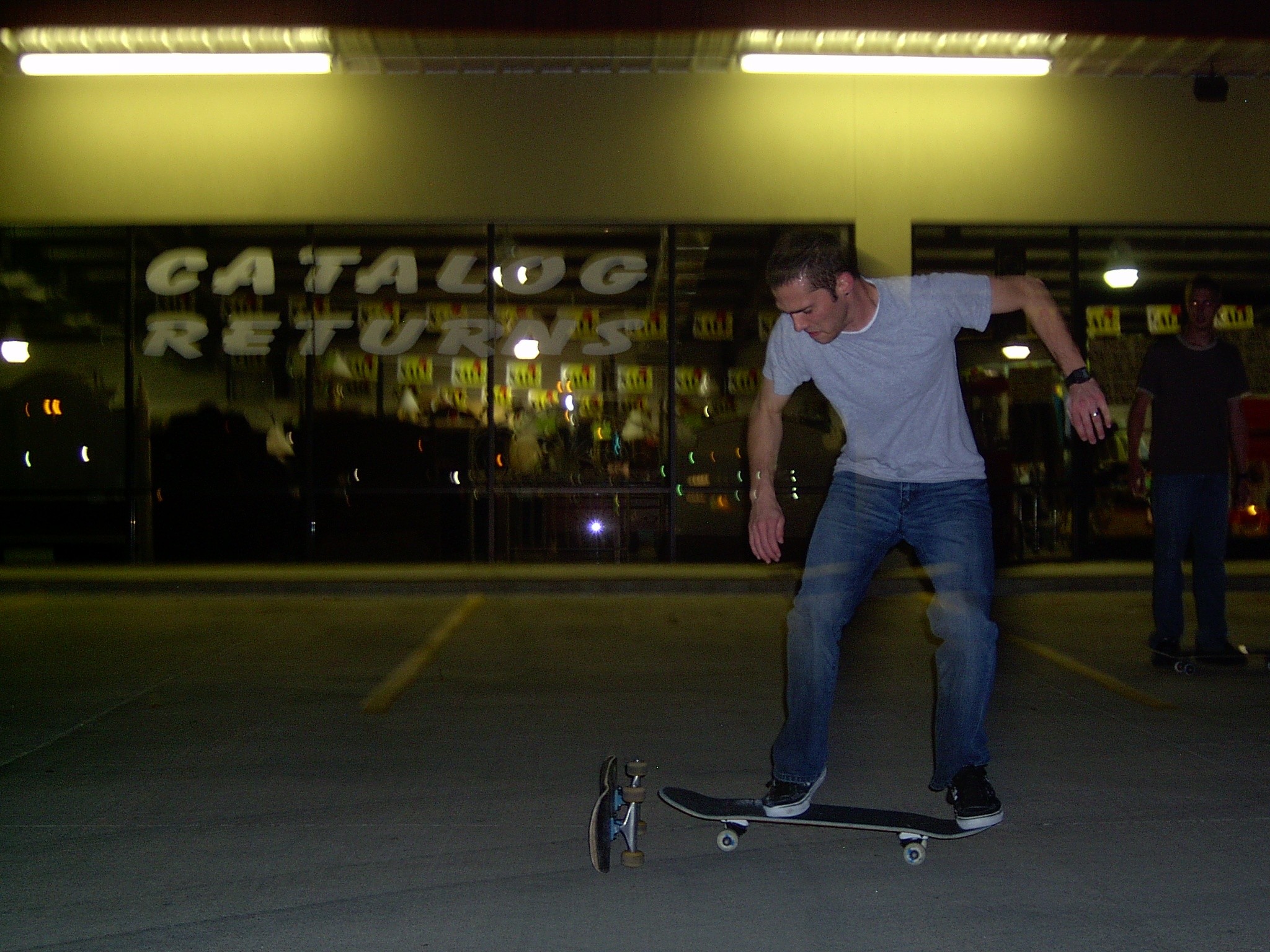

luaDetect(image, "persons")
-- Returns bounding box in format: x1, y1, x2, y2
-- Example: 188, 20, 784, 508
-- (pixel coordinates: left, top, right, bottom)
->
747, 227, 1112, 830
1127, 274, 1252, 662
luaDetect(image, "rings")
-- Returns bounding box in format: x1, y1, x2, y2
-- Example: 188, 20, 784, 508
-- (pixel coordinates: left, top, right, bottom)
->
1091, 412, 1099, 418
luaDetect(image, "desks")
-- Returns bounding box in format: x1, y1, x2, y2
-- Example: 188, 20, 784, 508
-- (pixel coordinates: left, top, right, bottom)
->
495, 472, 665, 564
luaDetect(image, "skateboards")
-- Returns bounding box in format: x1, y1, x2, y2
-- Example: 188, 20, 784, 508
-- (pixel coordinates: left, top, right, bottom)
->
1147, 644, 1270, 675
657, 787, 993, 865
589, 757, 648, 873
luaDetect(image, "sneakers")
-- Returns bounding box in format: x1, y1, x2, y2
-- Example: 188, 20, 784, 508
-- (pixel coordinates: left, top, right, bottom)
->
763, 762, 827, 818
949, 771, 1004, 829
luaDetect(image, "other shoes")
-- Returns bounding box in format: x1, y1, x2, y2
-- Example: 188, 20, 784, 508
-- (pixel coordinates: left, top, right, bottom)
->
1194, 640, 1245, 666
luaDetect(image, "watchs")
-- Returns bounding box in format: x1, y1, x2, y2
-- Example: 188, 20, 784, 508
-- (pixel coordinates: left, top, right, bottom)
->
1064, 366, 1096, 389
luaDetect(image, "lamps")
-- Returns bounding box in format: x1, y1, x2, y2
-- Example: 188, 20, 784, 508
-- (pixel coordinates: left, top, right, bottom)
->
20, 52, 331, 77
1001, 333, 1031, 360
0, 311, 31, 363
740, 54, 1052, 77
1103, 234, 1139, 289
